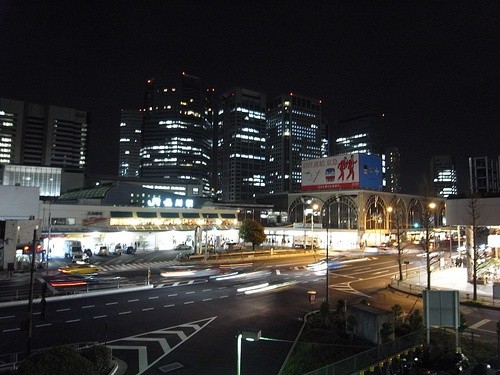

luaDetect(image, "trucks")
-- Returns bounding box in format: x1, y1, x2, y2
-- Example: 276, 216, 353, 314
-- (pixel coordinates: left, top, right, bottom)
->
64, 240, 82, 257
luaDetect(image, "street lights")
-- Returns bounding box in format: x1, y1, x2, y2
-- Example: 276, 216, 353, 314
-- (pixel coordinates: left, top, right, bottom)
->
233, 328, 261, 374
302, 194, 359, 325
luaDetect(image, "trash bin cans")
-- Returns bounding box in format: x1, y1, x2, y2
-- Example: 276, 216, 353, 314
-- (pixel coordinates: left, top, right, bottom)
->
493, 282, 500, 300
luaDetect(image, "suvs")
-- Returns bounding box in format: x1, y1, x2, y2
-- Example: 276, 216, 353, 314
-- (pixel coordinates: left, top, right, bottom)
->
225, 243, 243, 251
122, 246, 133, 254
108, 244, 122, 255
93, 246, 107, 256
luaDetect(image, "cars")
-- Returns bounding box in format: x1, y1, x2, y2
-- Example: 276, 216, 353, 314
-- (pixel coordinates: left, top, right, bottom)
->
71, 253, 90, 262
58, 261, 99, 274
175, 244, 191, 250
199, 245, 213, 253
